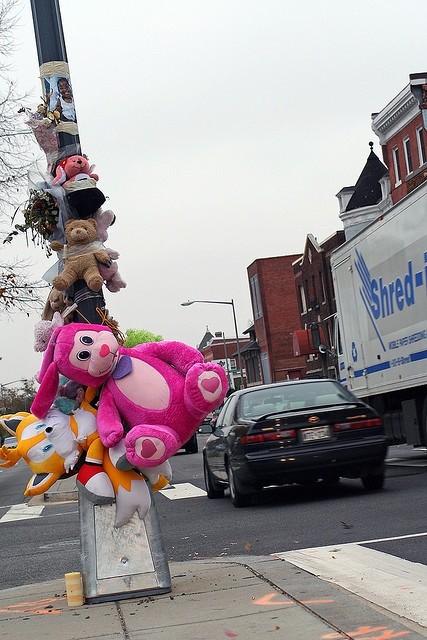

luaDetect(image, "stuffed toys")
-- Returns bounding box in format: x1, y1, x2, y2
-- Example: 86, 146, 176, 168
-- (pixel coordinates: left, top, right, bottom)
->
51, 154, 106, 213
49, 218, 110, 292
33, 311, 65, 384
39, 287, 70, 321
0, 386, 173, 528
53, 374, 85, 415
29, 323, 229, 467
92, 208, 127, 294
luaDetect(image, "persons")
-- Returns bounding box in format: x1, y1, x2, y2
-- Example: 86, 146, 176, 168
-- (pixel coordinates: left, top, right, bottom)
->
56, 77, 77, 122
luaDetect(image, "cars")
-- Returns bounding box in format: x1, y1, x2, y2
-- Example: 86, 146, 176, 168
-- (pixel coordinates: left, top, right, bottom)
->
197, 378, 386, 508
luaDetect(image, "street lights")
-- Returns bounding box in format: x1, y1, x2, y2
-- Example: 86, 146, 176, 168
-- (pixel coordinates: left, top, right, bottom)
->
181, 299, 246, 388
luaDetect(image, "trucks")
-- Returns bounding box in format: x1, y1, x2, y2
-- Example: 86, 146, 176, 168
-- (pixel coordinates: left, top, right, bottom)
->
306, 179, 426, 449
